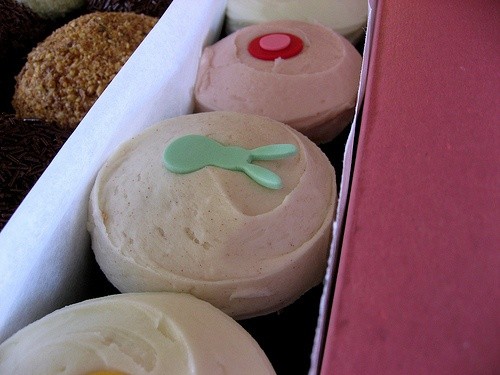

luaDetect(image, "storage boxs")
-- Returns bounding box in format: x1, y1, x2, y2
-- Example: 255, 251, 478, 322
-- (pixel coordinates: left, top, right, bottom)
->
0, 0, 500, 375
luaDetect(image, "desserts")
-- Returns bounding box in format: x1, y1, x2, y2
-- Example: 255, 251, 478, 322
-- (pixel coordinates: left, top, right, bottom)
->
0, 0, 374, 375
